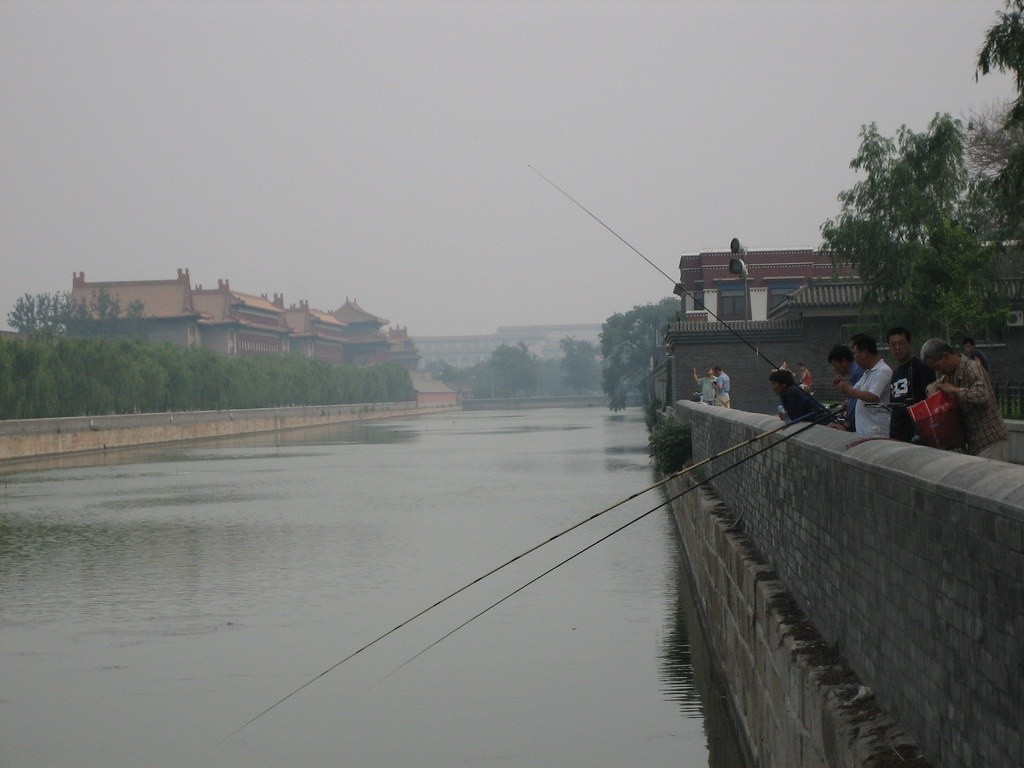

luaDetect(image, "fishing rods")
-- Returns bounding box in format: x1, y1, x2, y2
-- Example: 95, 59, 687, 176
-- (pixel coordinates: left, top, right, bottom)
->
526, 163, 850, 431
363, 402, 847, 694
226, 399, 844, 741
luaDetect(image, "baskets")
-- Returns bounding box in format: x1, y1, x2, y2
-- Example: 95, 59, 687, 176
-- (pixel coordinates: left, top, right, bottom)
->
914, 406, 965, 449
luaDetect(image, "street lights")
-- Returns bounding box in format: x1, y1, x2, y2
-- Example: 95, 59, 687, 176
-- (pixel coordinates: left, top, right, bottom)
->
728, 236, 750, 330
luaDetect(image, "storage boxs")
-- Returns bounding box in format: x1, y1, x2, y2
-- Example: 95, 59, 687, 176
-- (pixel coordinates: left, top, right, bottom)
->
908, 390, 966, 448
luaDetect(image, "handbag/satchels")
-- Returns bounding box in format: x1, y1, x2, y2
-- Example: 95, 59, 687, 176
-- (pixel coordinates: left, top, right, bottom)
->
906, 389, 967, 450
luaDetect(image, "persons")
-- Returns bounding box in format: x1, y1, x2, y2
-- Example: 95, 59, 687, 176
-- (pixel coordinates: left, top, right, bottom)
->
768, 370, 835, 426
889, 328, 937, 446
779, 362, 813, 389
691, 366, 730, 408
920, 336, 1009, 463
829, 333, 893, 438
